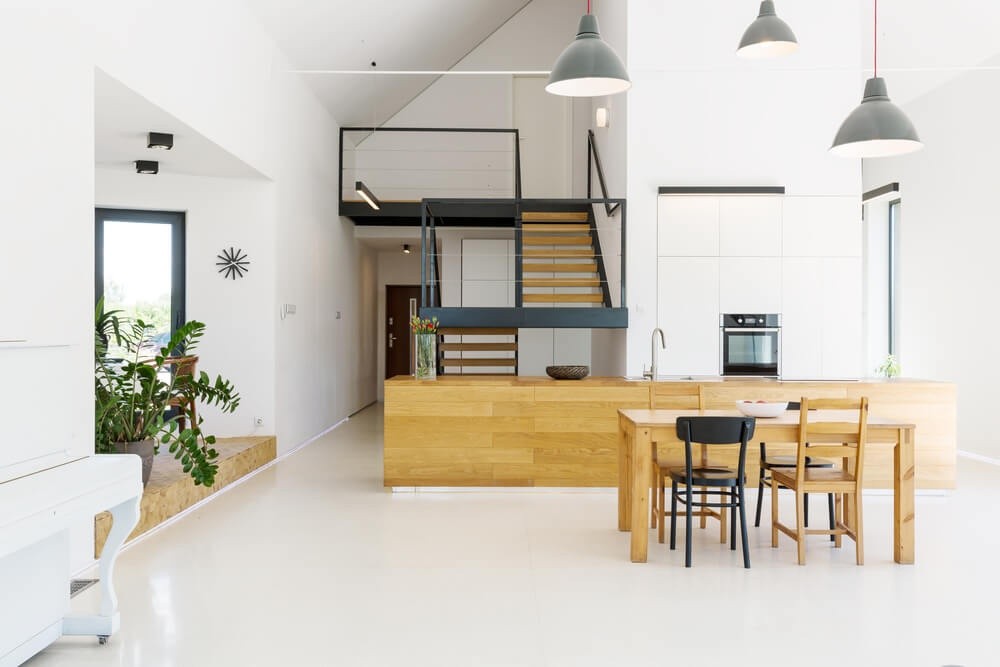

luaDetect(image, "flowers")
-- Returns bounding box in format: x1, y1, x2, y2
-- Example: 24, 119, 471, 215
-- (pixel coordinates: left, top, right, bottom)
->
409, 315, 440, 334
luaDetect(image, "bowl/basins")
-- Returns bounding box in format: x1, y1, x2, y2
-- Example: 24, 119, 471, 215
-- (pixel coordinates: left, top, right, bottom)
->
736, 400, 789, 417
546, 365, 589, 379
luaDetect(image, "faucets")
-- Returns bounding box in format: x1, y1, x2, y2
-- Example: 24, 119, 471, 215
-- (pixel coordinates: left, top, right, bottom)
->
643, 327, 666, 378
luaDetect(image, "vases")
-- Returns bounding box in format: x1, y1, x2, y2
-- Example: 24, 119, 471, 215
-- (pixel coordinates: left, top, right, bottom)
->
414, 335, 437, 379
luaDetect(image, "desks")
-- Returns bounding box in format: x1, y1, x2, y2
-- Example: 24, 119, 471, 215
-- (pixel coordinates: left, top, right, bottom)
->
616, 408, 917, 565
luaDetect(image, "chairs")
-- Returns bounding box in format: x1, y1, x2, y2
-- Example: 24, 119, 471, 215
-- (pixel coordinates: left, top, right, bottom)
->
769, 395, 868, 566
754, 402, 835, 541
670, 416, 756, 569
139, 354, 199, 447
649, 382, 727, 544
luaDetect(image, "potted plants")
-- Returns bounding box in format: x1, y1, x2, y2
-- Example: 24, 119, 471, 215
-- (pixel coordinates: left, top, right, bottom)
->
95, 297, 241, 491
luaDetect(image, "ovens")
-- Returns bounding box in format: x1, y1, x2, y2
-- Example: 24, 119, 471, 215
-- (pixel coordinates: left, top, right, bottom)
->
720, 314, 780, 376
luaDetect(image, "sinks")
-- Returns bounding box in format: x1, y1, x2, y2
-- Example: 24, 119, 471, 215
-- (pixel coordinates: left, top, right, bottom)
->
647, 375, 695, 380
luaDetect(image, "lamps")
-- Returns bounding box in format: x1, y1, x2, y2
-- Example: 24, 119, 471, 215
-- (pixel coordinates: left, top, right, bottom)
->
146, 132, 174, 150
737, 0, 797, 51
356, 182, 380, 210
136, 160, 159, 175
831, 0, 921, 157
545, 0, 630, 97
